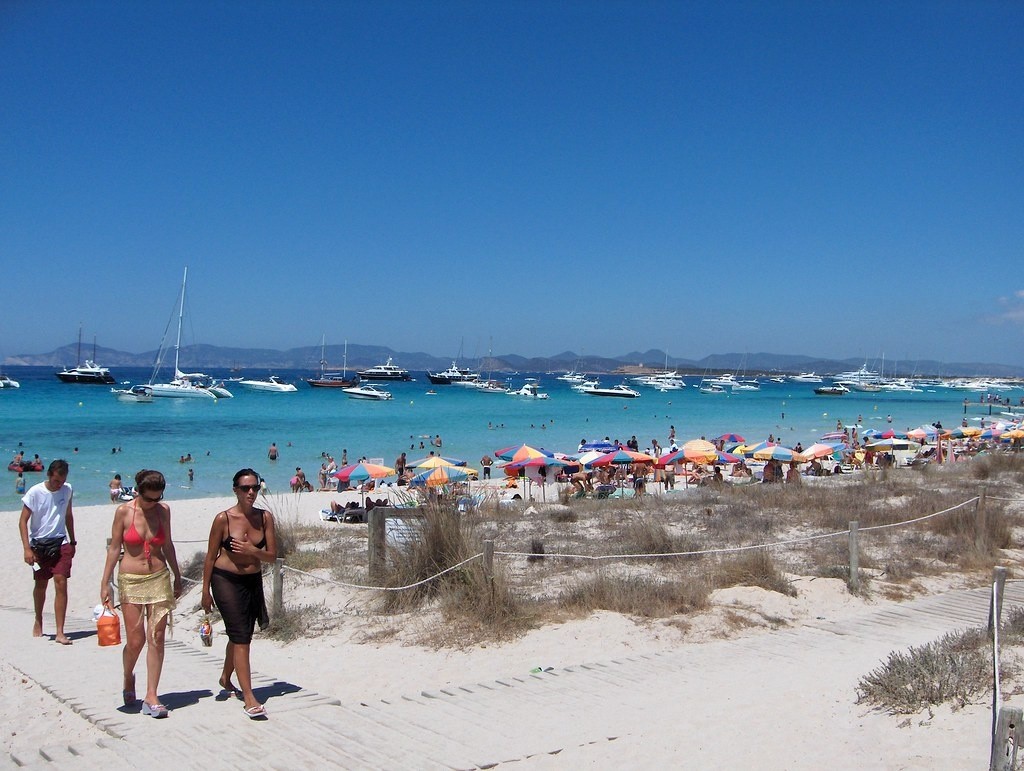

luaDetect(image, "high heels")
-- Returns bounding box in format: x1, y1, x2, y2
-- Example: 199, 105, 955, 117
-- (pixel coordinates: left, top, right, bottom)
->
122, 670, 136, 706
142, 700, 167, 718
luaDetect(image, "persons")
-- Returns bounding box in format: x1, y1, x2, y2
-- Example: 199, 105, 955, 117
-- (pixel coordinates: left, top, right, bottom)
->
35, 455, 41, 465
202, 468, 277, 718
108, 474, 125, 504
14, 451, 24, 464
267, 442, 279, 460
18, 460, 75, 645
188, 469, 194, 481
290, 467, 313, 493
319, 415, 988, 513
179, 453, 191, 464
260, 478, 268, 495
981, 392, 1002, 404
16, 474, 25, 494
1020, 396, 1024, 406
101, 469, 182, 718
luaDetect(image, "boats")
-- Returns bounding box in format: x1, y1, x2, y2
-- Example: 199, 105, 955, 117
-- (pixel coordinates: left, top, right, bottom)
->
118, 486, 139, 501
0, 375, 20, 388
813, 382, 849, 395
342, 381, 392, 401
584, 384, 642, 398
356, 354, 417, 383
118, 384, 153, 403
214, 376, 244, 382
239, 375, 298, 392
769, 372, 823, 383
7, 460, 44, 473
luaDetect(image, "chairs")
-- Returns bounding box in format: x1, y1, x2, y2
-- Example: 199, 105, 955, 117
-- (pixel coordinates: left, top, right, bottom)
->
595, 485, 616, 500
319, 510, 364, 524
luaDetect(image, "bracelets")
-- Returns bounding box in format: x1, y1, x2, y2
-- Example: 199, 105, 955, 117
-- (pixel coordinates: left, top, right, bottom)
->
70, 541, 77, 545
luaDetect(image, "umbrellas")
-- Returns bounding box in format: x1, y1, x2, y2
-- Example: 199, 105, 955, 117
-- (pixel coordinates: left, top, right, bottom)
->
334, 424, 1024, 507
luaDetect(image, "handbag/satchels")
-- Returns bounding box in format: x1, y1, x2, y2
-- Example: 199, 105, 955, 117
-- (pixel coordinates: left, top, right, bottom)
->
97, 602, 121, 646
31, 536, 67, 562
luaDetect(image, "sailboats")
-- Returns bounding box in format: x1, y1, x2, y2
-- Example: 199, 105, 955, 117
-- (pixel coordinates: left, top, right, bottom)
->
300, 333, 359, 387
554, 346, 603, 392
831, 349, 1024, 392
55, 322, 116, 384
137, 266, 234, 400
625, 347, 686, 390
693, 346, 760, 394
423, 335, 549, 399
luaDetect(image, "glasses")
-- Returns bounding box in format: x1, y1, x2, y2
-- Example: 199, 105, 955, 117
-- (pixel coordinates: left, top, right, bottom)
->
234, 485, 262, 492
141, 491, 164, 502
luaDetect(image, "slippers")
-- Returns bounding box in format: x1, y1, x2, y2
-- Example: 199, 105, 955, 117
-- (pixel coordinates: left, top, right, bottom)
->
220, 686, 242, 696
243, 704, 266, 717
